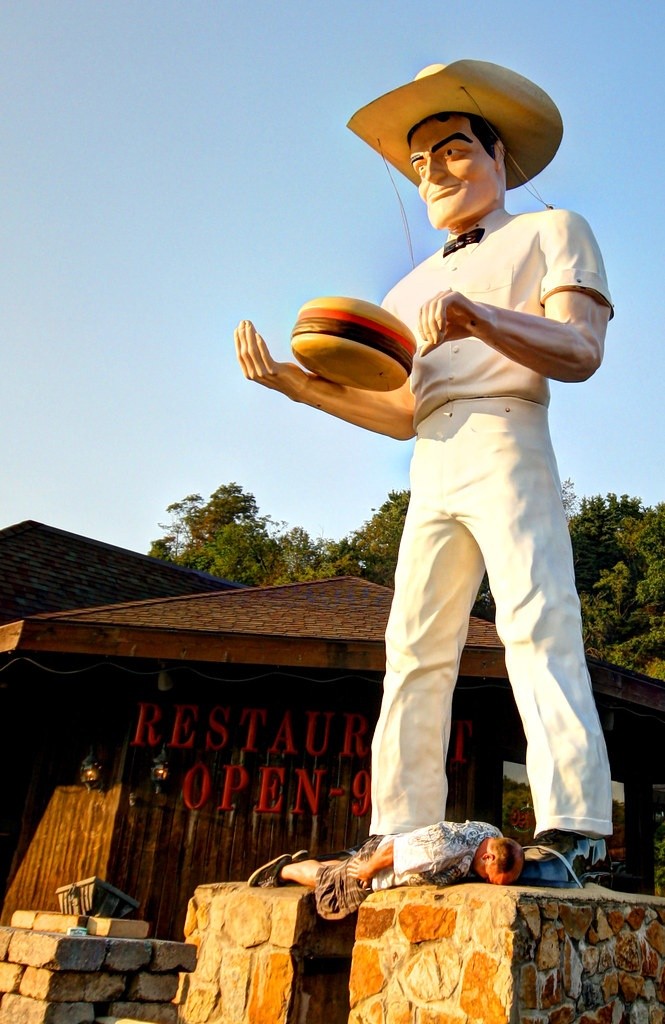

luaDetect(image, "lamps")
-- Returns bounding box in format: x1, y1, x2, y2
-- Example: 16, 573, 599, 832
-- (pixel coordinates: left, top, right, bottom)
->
149, 743, 170, 793
602, 709, 614, 731
79, 748, 111, 794
54, 875, 143, 918
158, 662, 175, 690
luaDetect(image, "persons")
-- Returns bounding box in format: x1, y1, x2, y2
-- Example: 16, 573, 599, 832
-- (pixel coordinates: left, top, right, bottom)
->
234, 61, 611, 885
246, 820, 523, 920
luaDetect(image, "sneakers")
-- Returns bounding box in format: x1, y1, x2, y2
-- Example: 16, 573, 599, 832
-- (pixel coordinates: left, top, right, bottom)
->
247, 853, 293, 888
293, 850, 309, 863
